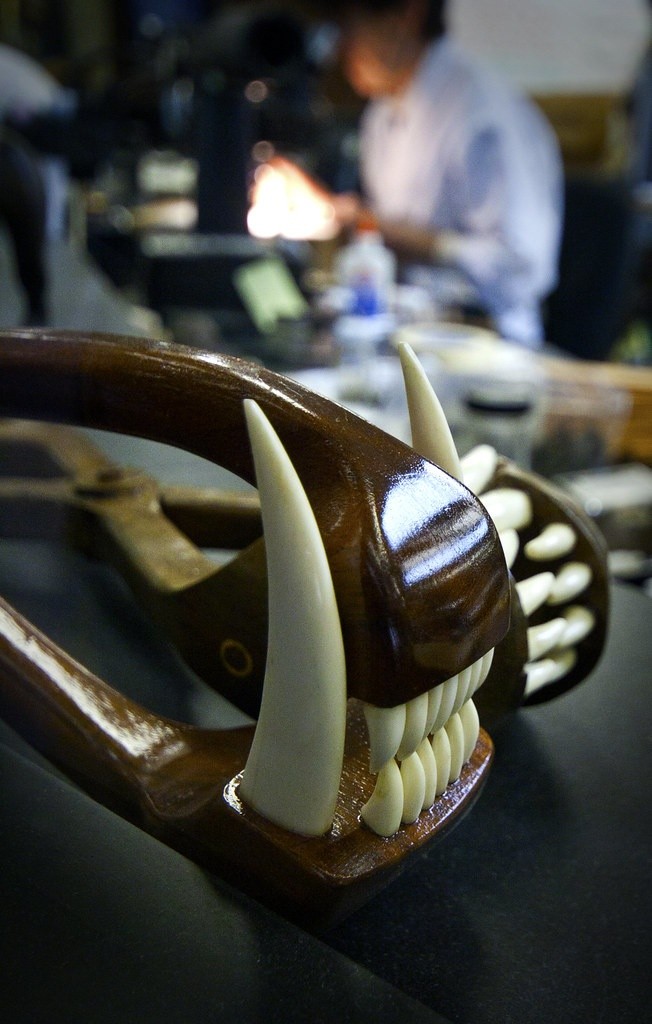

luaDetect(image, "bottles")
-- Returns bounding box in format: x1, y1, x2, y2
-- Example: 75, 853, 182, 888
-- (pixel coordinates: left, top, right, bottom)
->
330, 215, 395, 404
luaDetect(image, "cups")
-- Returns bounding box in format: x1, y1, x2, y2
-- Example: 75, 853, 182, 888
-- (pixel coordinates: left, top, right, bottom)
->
398, 321, 631, 478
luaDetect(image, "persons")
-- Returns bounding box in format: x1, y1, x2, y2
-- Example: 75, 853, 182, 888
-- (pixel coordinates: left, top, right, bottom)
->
333, 0, 563, 350
